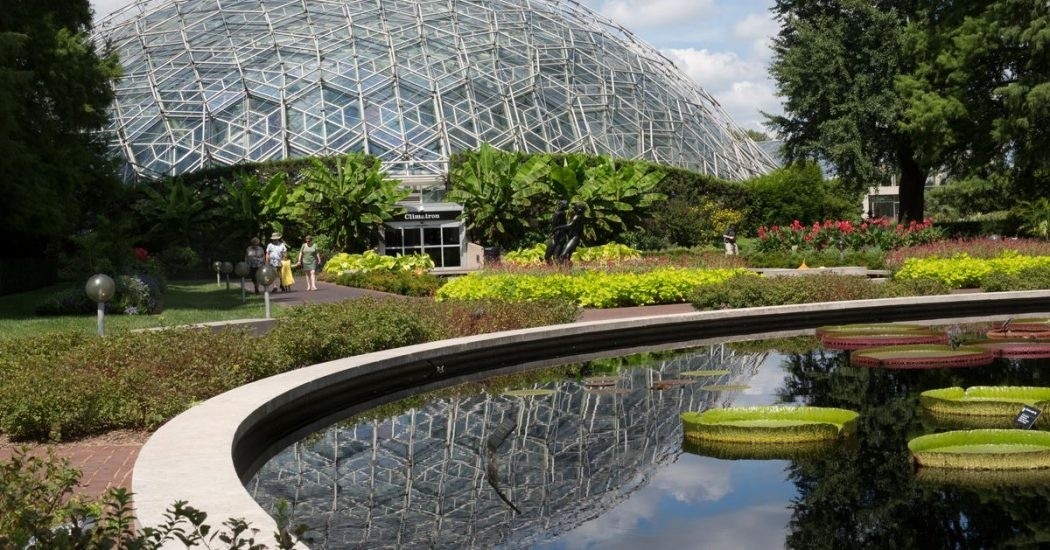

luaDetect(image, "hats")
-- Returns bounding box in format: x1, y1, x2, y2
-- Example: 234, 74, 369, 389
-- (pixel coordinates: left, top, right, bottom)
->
270, 233, 281, 239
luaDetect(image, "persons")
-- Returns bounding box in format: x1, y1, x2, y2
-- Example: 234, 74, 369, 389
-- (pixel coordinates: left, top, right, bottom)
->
544, 200, 587, 272
298, 235, 322, 292
244, 238, 266, 294
278, 252, 298, 293
723, 222, 739, 256
266, 232, 289, 294
